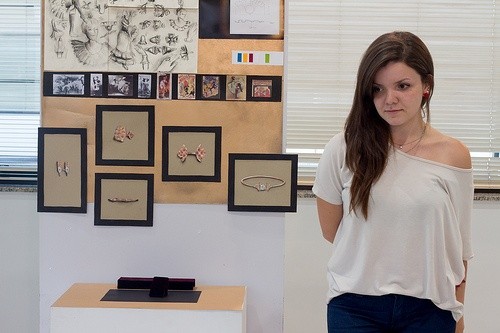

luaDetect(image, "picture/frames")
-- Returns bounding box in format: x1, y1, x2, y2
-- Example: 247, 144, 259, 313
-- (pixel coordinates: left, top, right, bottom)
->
163, 125, 221, 182
95, 173, 155, 227
37, 127, 87, 214
228, 153, 297, 213
95, 105, 154, 166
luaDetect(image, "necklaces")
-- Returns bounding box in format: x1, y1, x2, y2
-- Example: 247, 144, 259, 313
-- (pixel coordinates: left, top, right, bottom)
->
393, 134, 421, 149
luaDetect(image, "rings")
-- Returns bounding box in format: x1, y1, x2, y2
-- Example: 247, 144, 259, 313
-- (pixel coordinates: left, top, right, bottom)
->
241, 174, 285, 192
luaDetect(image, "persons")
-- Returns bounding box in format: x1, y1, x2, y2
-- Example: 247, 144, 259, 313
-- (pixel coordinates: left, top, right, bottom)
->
91, 75, 100, 96
141, 77, 151, 96
312, 31, 473, 332
112, 76, 130, 93
160, 75, 167, 98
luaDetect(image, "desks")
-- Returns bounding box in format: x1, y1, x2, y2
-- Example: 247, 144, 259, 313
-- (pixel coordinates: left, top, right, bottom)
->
49, 284, 247, 332
